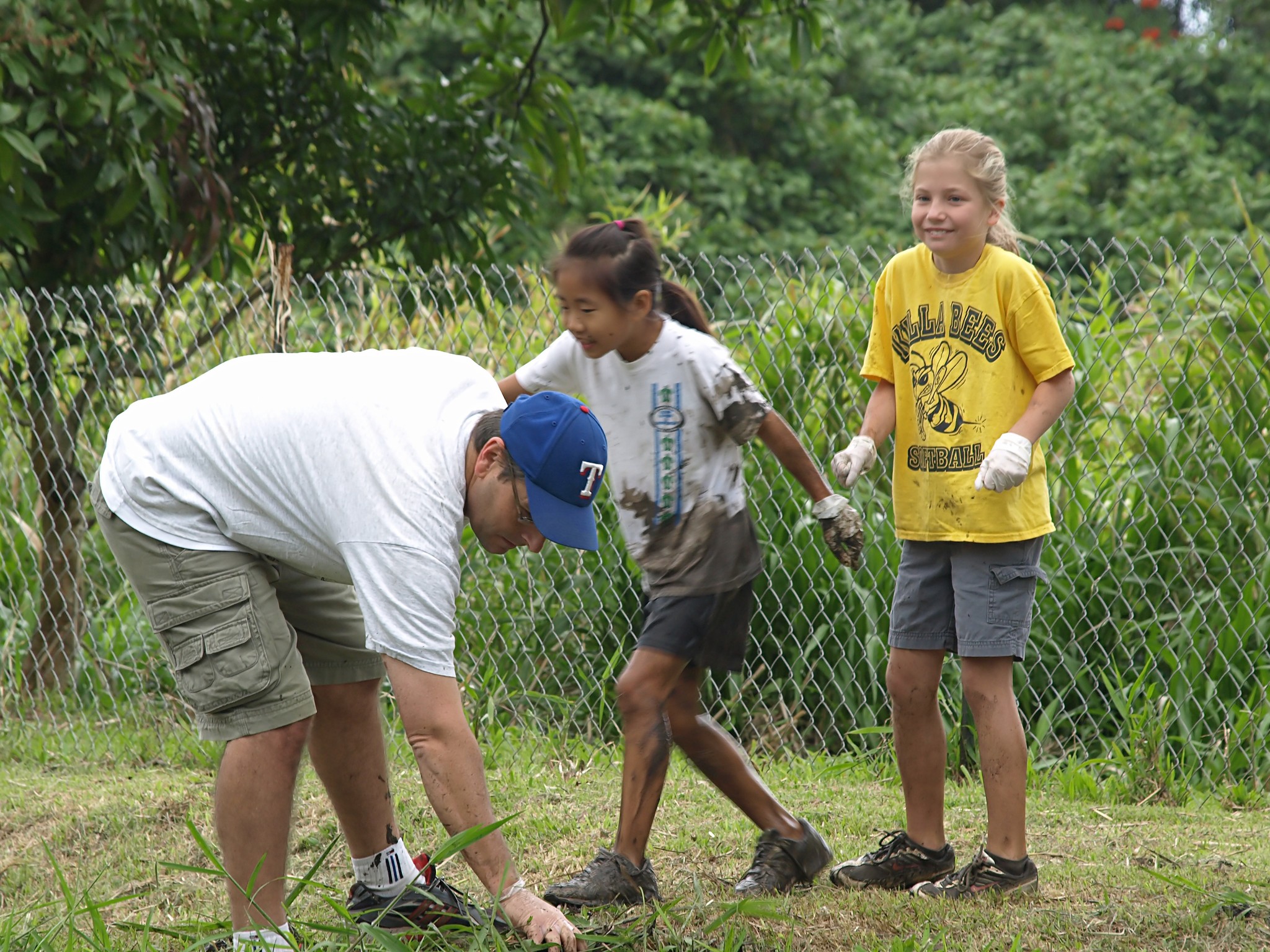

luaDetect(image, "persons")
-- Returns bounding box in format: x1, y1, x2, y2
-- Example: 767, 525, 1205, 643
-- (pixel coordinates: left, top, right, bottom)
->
831, 129, 1074, 901
90, 347, 607, 952
496, 221, 865, 908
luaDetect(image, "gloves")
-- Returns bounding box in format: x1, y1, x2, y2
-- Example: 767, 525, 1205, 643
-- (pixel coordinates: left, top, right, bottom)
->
812, 493, 867, 571
832, 435, 878, 489
976, 433, 1033, 492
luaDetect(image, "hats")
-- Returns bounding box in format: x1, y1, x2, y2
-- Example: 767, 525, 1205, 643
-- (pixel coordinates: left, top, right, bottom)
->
500, 392, 608, 549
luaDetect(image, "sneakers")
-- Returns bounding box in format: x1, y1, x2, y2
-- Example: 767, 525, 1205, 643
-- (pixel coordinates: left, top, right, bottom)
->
739, 819, 833, 895
910, 842, 1041, 901
347, 853, 513, 940
205, 919, 307, 952
545, 851, 660, 907
830, 828, 955, 890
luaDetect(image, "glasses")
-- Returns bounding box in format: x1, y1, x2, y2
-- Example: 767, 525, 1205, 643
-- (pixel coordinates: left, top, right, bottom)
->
504, 450, 535, 528
